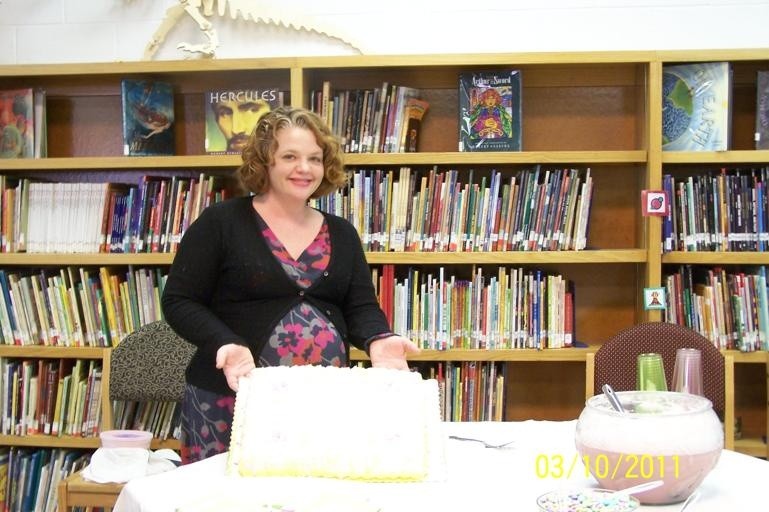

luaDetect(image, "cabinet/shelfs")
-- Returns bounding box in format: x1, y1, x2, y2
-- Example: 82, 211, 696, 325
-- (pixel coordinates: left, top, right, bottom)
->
0, 48, 769, 512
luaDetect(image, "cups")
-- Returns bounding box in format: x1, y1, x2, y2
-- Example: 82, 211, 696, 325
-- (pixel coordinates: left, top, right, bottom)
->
635, 348, 703, 391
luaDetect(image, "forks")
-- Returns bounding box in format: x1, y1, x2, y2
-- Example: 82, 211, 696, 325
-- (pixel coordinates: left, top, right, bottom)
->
450, 435, 515, 448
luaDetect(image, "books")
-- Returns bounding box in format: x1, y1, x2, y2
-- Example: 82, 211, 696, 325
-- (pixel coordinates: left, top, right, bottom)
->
1, 359, 103, 438
0, 266, 168, 347
661, 264, 768, 352
663, 61, 733, 153
1, 447, 104, 512
1, 175, 231, 254
113, 397, 183, 441
661, 166, 768, 252
458, 71, 522, 153
351, 360, 506, 421
1, 88, 48, 159
755, 71, 768, 149
122, 79, 176, 155
205, 91, 283, 155
308, 165, 593, 253
371, 263, 575, 350
308, 80, 427, 153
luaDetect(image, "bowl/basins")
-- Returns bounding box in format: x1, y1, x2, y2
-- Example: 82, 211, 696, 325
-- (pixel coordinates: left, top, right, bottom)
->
101, 429, 153, 449
574, 391, 724, 505
537, 485, 640, 512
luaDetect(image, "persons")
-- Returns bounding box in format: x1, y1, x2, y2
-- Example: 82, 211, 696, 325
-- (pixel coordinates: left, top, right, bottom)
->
160, 107, 420, 466
